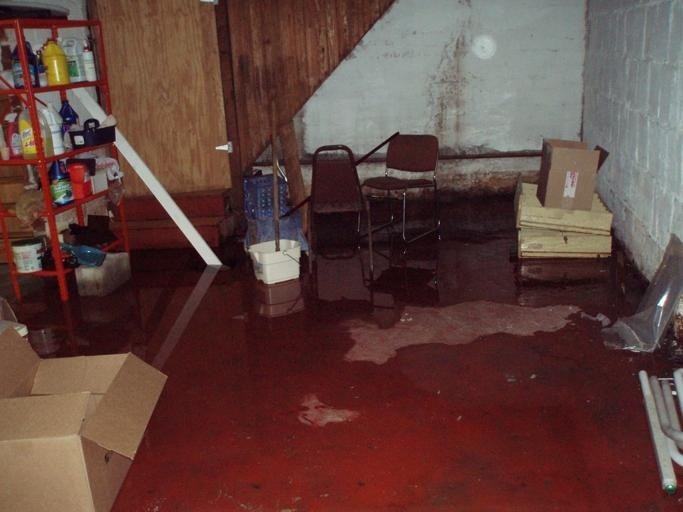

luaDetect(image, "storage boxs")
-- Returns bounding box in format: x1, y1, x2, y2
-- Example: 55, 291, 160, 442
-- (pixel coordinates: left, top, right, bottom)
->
536, 138, 609, 210
0, 326, 170, 510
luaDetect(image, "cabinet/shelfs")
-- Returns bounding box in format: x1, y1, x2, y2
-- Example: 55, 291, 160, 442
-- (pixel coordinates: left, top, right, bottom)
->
0, 18, 130, 306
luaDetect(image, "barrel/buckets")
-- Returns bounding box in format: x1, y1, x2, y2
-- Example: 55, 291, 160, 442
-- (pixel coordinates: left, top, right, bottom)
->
254, 280, 305, 317
29, 328, 60, 355
11, 240, 43, 274
249, 238, 302, 284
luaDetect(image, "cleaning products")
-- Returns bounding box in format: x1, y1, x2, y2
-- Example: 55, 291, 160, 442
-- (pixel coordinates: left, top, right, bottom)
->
0, 37, 96, 207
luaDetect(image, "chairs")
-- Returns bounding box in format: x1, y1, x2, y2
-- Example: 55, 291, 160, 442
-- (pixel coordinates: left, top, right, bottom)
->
305, 134, 444, 288
